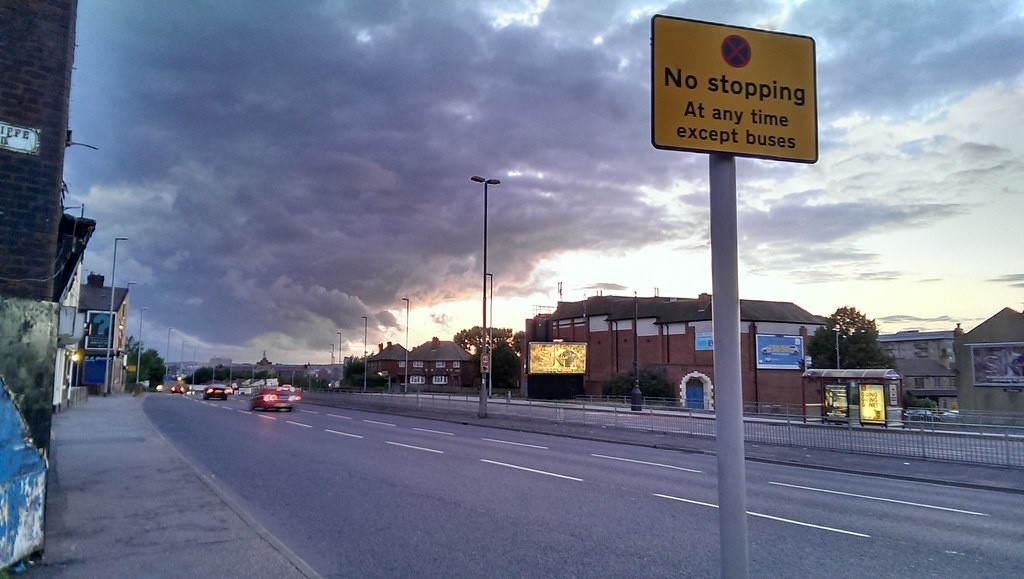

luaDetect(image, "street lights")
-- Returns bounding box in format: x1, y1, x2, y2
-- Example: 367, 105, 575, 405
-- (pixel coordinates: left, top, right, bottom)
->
136, 308, 149, 382
164, 327, 175, 387
193, 349, 198, 389
336, 333, 341, 388
180, 341, 188, 379
402, 298, 410, 393
127, 281, 137, 289
102, 237, 128, 396
486, 273, 493, 397
361, 317, 367, 392
470, 176, 501, 418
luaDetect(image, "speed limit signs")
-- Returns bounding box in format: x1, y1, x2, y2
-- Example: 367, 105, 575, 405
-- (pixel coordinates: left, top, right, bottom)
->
481, 354, 490, 364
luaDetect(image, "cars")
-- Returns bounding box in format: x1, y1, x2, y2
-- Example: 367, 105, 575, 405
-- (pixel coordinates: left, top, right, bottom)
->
171, 385, 183, 395
203, 384, 228, 400
248, 388, 295, 412
904, 410, 941, 427
